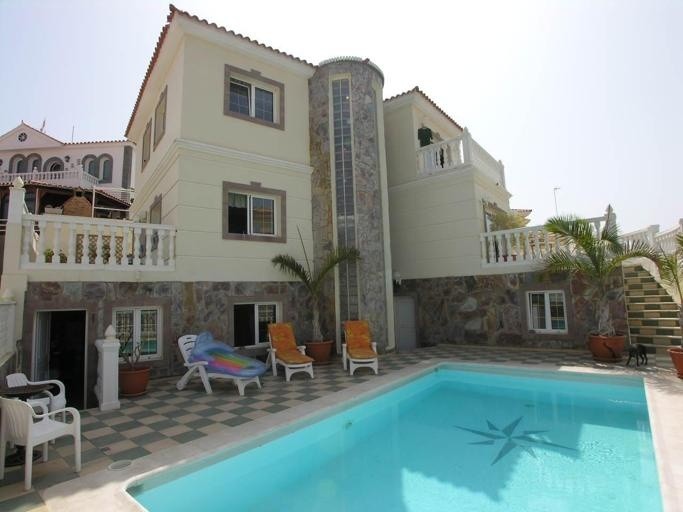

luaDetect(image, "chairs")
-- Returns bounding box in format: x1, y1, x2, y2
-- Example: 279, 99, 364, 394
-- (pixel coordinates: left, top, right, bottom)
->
176, 335, 262, 395
0, 372, 84, 491
340, 318, 380, 378
264, 322, 316, 384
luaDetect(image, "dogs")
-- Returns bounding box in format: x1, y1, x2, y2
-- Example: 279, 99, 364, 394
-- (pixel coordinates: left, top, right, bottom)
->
622, 343, 647, 366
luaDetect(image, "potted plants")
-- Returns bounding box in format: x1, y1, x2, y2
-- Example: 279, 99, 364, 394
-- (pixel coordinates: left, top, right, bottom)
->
116, 327, 151, 395
44, 248, 55, 263
536, 211, 682, 379
268, 246, 364, 365
